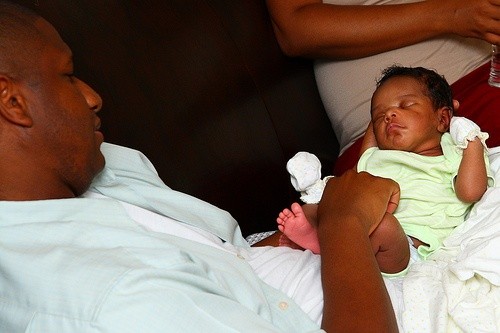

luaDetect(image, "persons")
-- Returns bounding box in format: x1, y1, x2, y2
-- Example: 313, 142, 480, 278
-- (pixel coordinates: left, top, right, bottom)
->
1, 1, 500, 333
268, 1, 500, 181
277, 65, 495, 279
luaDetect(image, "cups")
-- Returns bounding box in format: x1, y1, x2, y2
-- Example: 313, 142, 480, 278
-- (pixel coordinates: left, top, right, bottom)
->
488, 45, 500, 87
